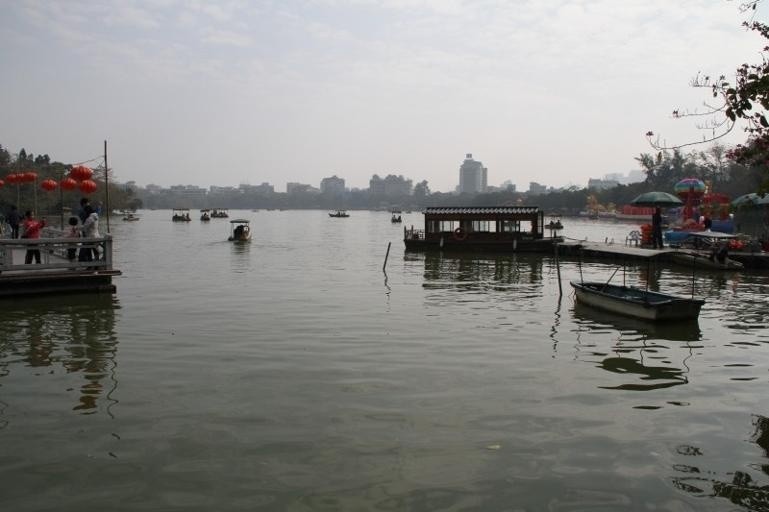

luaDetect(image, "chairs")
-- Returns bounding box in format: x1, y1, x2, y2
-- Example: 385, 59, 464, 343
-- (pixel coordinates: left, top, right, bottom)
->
625, 230, 642, 246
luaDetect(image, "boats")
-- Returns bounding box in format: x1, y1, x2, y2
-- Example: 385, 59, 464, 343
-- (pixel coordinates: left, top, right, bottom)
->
227, 219, 252, 241
200, 208, 210, 222
211, 207, 229, 217
403, 205, 564, 253
123, 211, 140, 222
328, 209, 349, 217
665, 229, 746, 271
391, 210, 402, 223
543, 219, 564, 230
172, 207, 192, 222
569, 278, 706, 321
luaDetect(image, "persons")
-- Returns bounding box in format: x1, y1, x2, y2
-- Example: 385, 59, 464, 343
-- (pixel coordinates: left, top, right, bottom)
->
550, 220, 555, 225
702, 212, 713, 232
651, 207, 665, 249
556, 220, 560, 224
5, 199, 101, 269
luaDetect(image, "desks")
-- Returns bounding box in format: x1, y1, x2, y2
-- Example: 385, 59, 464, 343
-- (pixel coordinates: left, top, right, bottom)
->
689, 231, 738, 252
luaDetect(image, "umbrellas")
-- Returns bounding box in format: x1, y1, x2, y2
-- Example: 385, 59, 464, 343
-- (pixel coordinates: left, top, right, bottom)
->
629, 191, 683, 225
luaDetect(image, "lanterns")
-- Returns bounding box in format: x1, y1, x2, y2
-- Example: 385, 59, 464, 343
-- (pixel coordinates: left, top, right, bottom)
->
0, 165, 96, 194
